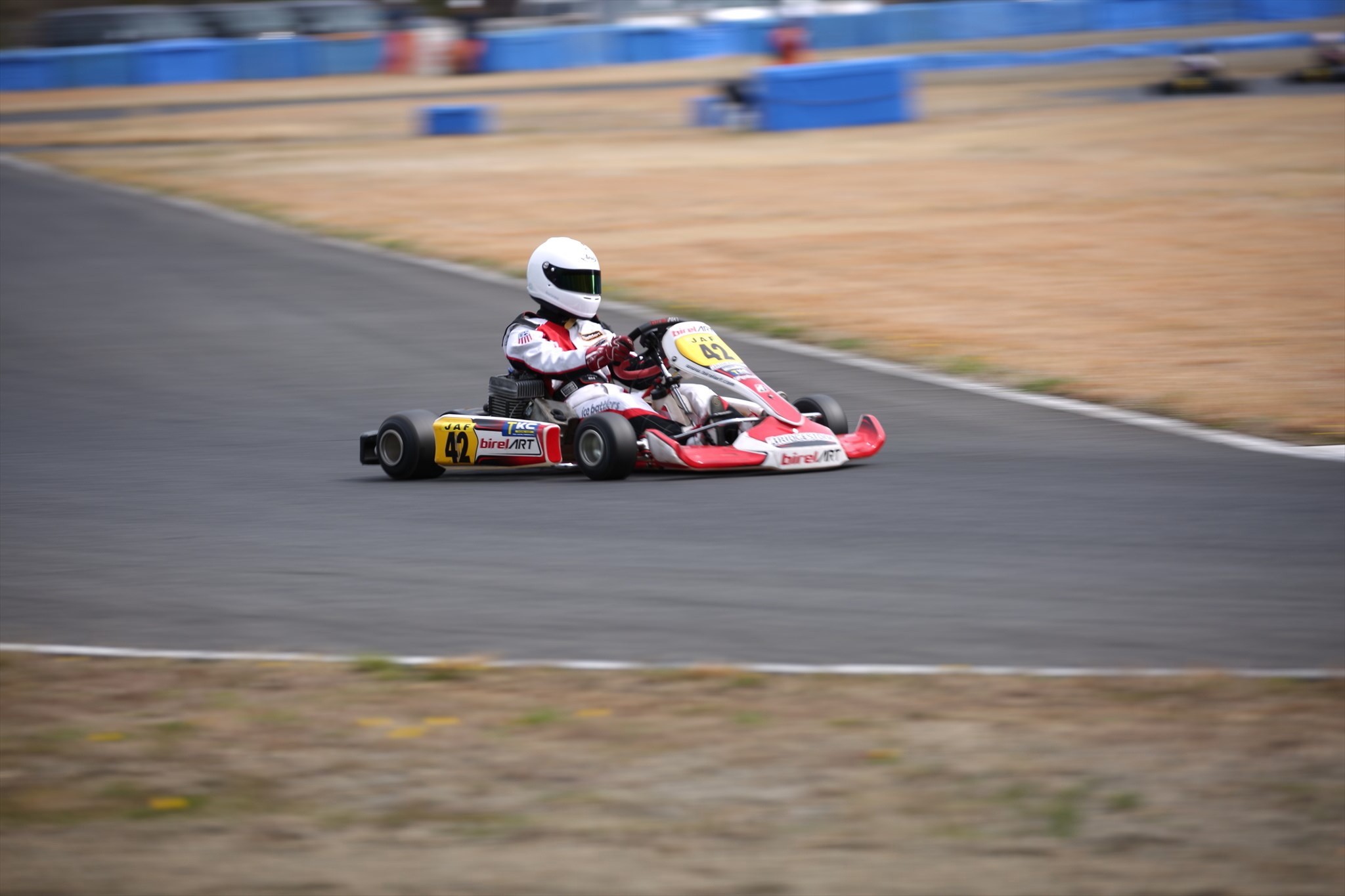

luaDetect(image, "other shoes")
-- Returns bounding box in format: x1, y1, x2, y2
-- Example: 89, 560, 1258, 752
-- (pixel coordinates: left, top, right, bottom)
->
700, 395, 726, 446
766, 392, 788, 416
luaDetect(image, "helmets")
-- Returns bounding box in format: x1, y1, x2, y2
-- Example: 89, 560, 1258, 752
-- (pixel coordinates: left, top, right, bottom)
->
527, 237, 601, 320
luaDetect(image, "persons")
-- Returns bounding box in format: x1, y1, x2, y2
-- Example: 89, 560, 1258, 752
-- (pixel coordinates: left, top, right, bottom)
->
502, 236, 752, 445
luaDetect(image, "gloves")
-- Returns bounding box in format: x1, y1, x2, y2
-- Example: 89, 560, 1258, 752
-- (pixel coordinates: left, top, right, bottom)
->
585, 336, 634, 374
